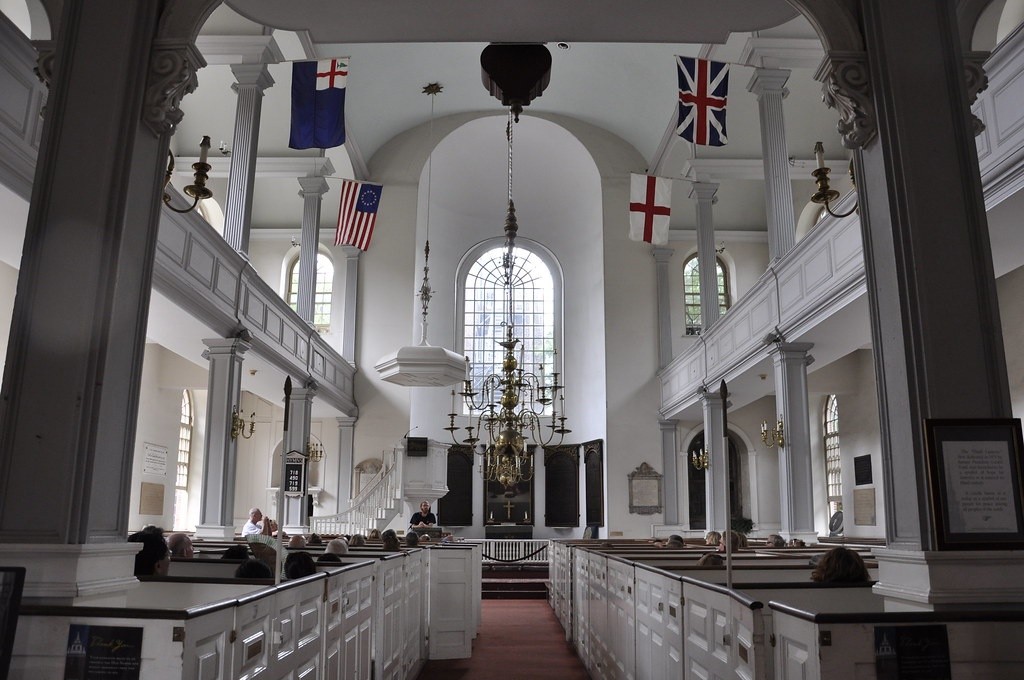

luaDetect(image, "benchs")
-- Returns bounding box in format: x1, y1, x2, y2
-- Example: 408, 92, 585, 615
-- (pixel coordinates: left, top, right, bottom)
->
548, 539, 881, 680
135, 535, 485, 680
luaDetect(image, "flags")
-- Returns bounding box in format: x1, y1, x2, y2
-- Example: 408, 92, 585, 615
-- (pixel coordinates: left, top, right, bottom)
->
335, 179, 383, 251
676, 56, 730, 146
288, 57, 349, 150
629, 173, 673, 245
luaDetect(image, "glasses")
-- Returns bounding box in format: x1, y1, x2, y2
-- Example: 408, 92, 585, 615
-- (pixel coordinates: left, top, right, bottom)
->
812, 571, 820, 576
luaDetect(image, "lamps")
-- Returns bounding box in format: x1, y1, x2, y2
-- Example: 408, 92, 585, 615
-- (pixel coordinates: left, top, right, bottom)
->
696, 386, 706, 395
716, 241, 725, 253
375, 84, 472, 389
761, 418, 784, 448
762, 333, 781, 346
242, 329, 254, 342
291, 233, 301, 247
810, 142, 859, 218
781, 88, 790, 100
162, 136, 212, 213
308, 442, 323, 463
310, 381, 319, 391
692, 447, 709, 470
713, 196, 718, 205
442, 41, 575, 500
231, 405, 255, 443
727, 401, 734, 408
219, 140, 231, 158
201, 350, 210, 360
806, 355, 815, 366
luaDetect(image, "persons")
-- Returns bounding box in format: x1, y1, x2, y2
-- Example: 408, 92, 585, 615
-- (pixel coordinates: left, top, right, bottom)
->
128, 525, 169, 579
166, 533, 193, 557
410, 500, 436, 528
241, 508, 272, 537
811, 546, 869, 589
221, 528, 453, 580
658, 529, 806, 567
